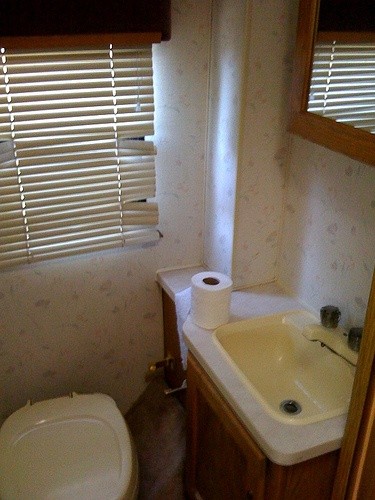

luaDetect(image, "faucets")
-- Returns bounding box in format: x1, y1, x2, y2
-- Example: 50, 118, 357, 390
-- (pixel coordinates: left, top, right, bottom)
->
302, 322, 358, 367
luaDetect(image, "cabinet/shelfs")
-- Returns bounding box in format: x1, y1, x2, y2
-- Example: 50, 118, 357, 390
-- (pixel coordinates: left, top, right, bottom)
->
185, 350, 267, 500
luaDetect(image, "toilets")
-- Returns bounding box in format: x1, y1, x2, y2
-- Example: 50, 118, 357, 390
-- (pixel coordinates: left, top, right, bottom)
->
1, 390, 141, 499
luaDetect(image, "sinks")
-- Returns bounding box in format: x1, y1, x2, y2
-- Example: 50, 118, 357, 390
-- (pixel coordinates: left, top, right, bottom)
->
212, 309, 358, 426
